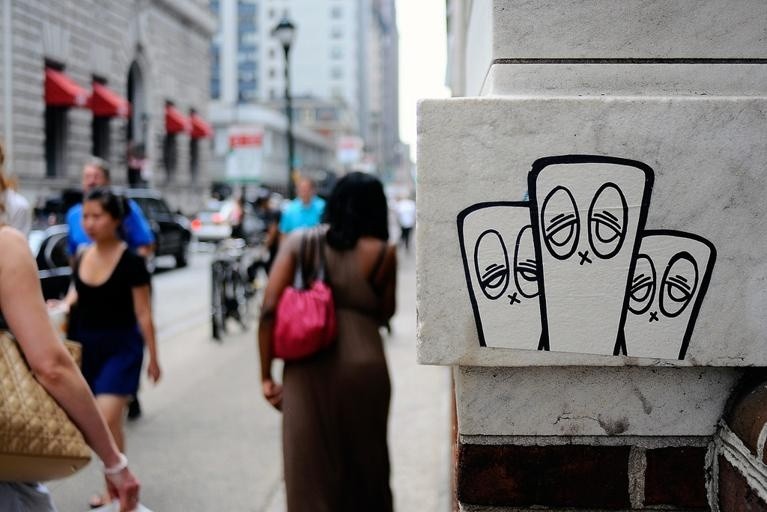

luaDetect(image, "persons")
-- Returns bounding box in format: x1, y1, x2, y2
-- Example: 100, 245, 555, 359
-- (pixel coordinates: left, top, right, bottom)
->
1, 136, 144, 512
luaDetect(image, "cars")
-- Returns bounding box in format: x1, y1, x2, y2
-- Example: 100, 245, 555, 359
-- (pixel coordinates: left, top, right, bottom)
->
194, 202, 241, 242
124, 188, 195, 267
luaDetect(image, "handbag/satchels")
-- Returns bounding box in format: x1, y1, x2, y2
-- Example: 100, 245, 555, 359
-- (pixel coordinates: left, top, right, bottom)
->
272, 282, 336, 359
0, 331, 91, 482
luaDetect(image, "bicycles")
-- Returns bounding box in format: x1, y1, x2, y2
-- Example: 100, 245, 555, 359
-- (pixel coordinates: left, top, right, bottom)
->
212, 258, 250, 337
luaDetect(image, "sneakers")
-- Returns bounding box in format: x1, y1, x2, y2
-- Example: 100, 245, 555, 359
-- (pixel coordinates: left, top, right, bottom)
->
127, 404, 142, 421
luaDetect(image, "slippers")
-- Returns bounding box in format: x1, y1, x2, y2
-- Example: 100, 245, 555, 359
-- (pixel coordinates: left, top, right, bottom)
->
89, 492, 111, 507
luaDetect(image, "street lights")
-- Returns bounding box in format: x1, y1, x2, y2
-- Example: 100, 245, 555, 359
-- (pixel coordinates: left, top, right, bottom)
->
271, 11, 297, 196
